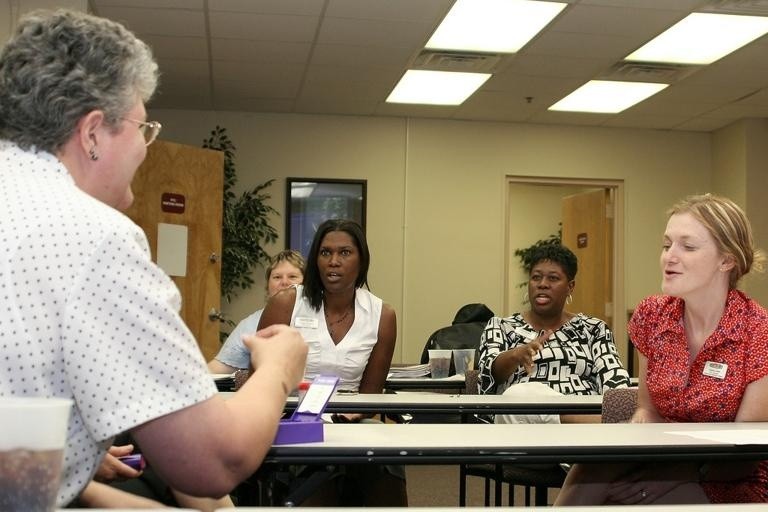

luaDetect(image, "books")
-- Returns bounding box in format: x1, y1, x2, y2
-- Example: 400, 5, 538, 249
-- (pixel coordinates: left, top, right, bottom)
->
389, 363, 431, 379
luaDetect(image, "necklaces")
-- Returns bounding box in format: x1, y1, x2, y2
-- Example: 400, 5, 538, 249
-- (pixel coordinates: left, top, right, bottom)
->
323, 298, 354, 339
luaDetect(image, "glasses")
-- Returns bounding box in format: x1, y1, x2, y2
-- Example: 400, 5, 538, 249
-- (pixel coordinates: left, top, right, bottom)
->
120, 116, 162, 146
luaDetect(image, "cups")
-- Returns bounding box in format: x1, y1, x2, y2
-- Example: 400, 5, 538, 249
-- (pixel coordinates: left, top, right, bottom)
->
428, 349, 452, 380
450, 349, 477, 375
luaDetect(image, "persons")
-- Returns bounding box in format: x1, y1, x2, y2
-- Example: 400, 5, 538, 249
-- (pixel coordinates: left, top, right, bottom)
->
248, 219, 410, 506
476, 242, 632, 471
63, 429, 235, 510
2, 4, 309, 509
546, 192, 768, 504
205, 249, 308, 375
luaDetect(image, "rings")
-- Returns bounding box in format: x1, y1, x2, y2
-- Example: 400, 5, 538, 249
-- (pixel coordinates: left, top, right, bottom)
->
640, 488, 649, 498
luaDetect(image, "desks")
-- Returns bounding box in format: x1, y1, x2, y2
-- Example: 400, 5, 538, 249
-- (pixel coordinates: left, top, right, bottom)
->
216, 364, 768, 506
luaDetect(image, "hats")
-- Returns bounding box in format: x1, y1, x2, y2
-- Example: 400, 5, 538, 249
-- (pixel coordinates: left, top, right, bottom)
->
452, 304, 494, 325
